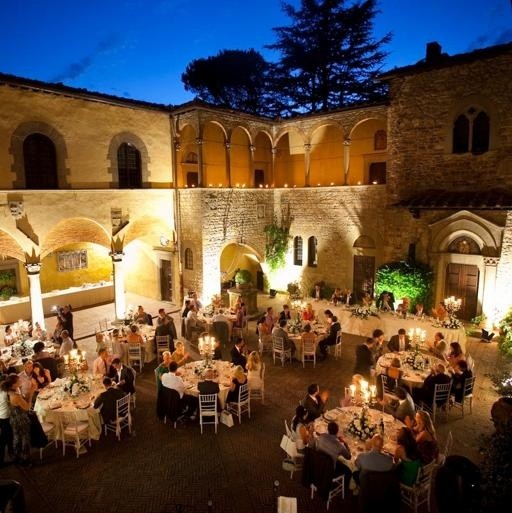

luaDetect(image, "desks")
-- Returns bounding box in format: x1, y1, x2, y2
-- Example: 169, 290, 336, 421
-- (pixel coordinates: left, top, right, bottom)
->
257, 290, 468, 355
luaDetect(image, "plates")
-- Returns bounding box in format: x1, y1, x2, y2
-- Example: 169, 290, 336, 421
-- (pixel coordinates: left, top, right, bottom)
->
324, 411, 337, 420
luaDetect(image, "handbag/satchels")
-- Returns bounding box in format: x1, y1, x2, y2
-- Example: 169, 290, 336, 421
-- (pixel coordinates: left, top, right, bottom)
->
280, 435, 298, 458
219, 410, 234, 427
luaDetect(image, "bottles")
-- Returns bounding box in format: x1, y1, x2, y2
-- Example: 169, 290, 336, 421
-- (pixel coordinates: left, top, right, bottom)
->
379, 418, 384, 434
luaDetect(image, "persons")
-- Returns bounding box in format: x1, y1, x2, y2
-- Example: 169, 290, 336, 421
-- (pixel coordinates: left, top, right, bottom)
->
181, 291, 246, 335
112, 304, 177, 366
255, 303, 341, 363
0, 304, 135, 466
156, 338, 248, 428
311, 282, 449, 324
289, 375, 440, 513
355, 328, 473, 411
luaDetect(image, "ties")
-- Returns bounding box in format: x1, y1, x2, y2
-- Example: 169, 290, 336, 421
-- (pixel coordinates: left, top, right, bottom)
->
103, 359, 107, 376
400, 339, 403, 351
